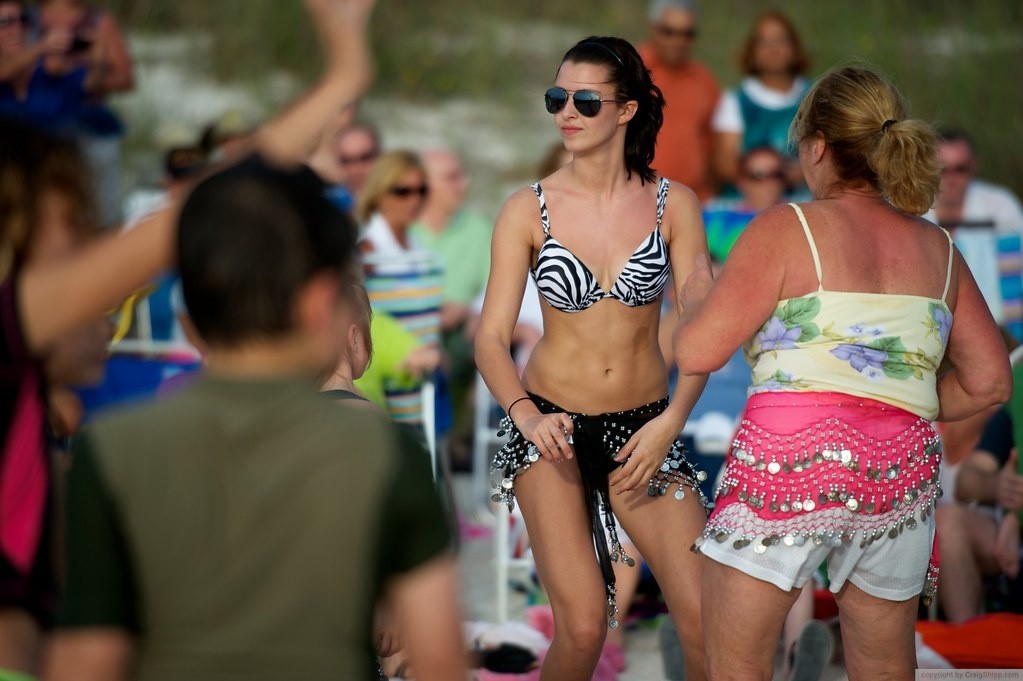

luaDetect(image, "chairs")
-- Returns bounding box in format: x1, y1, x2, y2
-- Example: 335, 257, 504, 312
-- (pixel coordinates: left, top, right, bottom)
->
471, 370, 507, 500
362, 248, 446, 485
494, 492, 534, 623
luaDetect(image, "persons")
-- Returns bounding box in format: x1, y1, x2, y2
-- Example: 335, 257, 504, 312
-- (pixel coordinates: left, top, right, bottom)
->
40, 147, 466, 681
472, 33, 712, 681
0, 0, 374, 681
0, 0, 1023, 681
672, 59, 1012, 681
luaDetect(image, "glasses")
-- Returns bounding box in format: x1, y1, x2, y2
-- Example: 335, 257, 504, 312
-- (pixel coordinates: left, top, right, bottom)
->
544, 87, 630, 118
336, 151, 378, 167
655, 22, 696, 41
0, 14, 35, 28
388, 186, 428, 199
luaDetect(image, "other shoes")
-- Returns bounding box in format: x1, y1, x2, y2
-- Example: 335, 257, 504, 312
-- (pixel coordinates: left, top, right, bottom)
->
788, 619, 833, 681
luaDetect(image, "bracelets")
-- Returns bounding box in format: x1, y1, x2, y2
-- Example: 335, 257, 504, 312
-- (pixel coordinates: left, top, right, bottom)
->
508, 397, 533, 418
